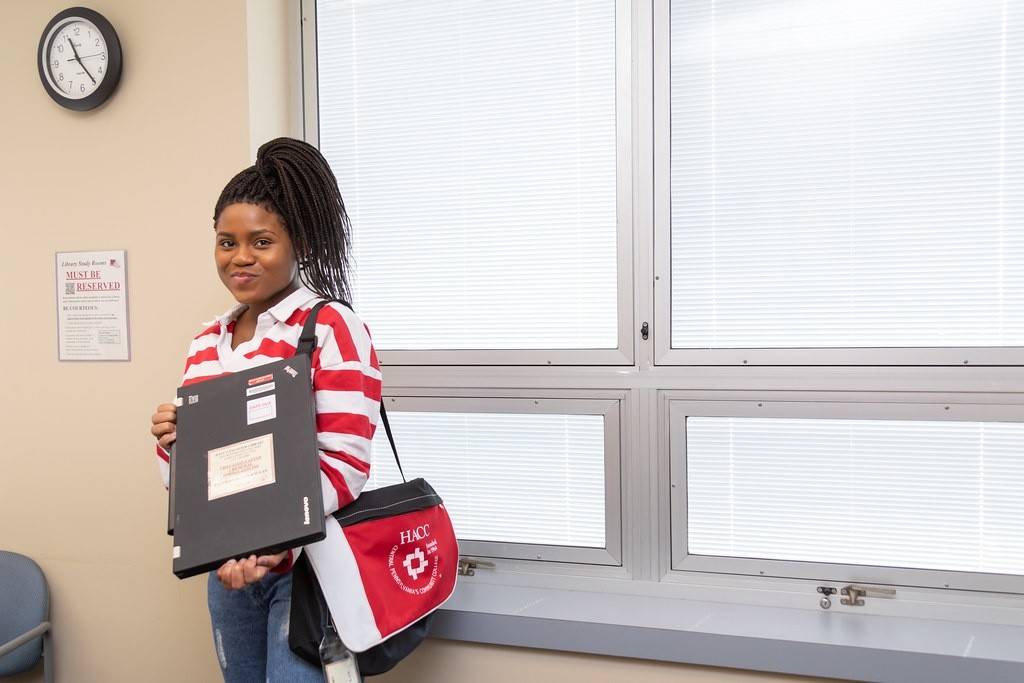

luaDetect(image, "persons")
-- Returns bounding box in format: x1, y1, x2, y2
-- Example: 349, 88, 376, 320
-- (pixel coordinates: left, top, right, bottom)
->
151, 137, 381, 683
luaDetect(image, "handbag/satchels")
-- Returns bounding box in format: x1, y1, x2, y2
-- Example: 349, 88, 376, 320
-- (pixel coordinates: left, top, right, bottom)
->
287, 476, 460, 678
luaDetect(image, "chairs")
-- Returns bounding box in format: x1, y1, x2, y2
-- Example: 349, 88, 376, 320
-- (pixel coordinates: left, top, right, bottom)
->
0, 551, 52, 683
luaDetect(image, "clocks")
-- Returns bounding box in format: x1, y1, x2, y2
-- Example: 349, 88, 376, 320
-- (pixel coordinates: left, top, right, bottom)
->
37, 6, 123, 112
